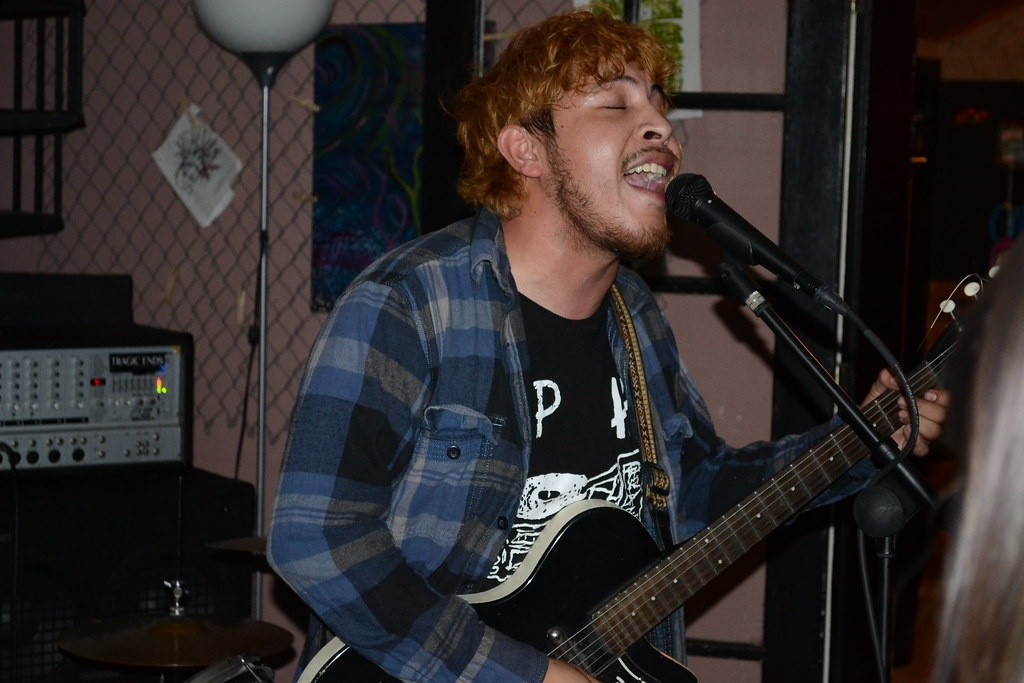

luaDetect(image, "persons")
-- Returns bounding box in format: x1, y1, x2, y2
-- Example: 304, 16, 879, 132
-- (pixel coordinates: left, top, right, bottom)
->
266, 2, 1023, 683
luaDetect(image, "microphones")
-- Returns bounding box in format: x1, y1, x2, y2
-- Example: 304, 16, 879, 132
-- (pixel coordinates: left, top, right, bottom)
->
666, 172, 843, 312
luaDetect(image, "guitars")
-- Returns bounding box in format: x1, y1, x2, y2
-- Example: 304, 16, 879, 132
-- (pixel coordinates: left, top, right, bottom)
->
293, 260, 1013, 683
